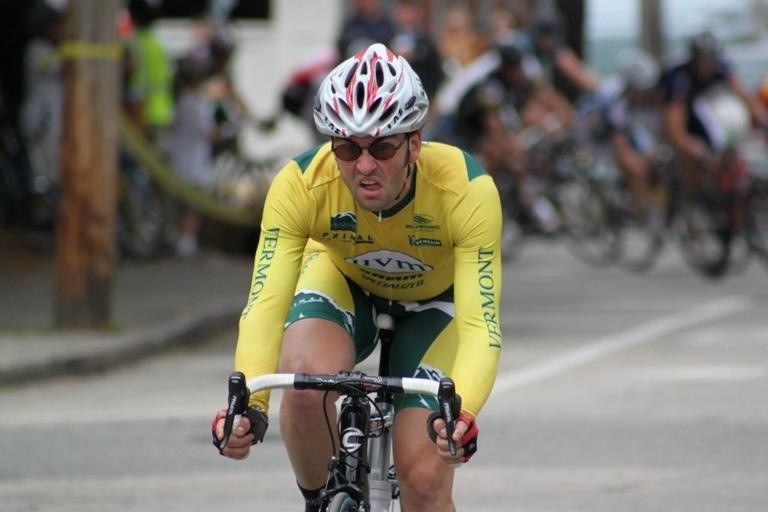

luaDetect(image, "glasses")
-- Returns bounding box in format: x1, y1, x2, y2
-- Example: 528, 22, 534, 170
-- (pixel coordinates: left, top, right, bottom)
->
328, 133, 409, 162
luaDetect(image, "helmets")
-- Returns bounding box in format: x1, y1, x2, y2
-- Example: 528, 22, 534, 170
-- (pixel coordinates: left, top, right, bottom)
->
312, 42, 429, 140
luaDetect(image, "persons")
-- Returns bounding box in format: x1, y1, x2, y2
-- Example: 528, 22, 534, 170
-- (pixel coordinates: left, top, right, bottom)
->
1, 0, 322, 268
338, 1, 767, 230
212, 43, 503, 512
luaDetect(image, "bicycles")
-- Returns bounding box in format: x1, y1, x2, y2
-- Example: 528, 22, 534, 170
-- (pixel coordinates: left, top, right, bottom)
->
219, 370, 460, 512
436, 77, 768, 283
